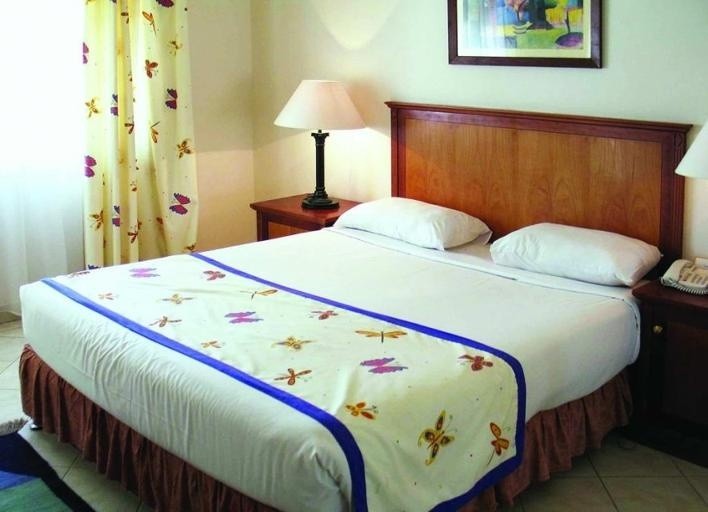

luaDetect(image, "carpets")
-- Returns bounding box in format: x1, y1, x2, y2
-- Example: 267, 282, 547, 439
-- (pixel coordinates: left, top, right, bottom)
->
2, 432, 98, 512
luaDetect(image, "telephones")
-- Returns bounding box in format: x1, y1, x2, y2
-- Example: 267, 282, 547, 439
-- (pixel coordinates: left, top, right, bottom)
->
661, 258, 708, 292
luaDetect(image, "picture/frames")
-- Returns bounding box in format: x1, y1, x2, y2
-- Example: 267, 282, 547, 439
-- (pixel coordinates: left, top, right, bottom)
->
447, 0, 604, 66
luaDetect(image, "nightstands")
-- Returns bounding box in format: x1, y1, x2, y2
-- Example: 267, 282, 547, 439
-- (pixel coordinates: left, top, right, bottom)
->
625, 278, 707, 469
249, 194, 360, 242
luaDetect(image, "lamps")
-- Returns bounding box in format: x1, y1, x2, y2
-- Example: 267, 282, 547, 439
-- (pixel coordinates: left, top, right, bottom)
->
272, 79, 366, 209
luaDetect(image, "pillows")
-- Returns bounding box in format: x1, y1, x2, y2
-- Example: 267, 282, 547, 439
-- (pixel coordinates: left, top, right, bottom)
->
334, 198, 489, 252
490, 218, 665, 288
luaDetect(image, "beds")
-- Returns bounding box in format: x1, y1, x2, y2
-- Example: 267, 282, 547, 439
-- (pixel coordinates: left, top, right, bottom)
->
18, 101, 695, 503
